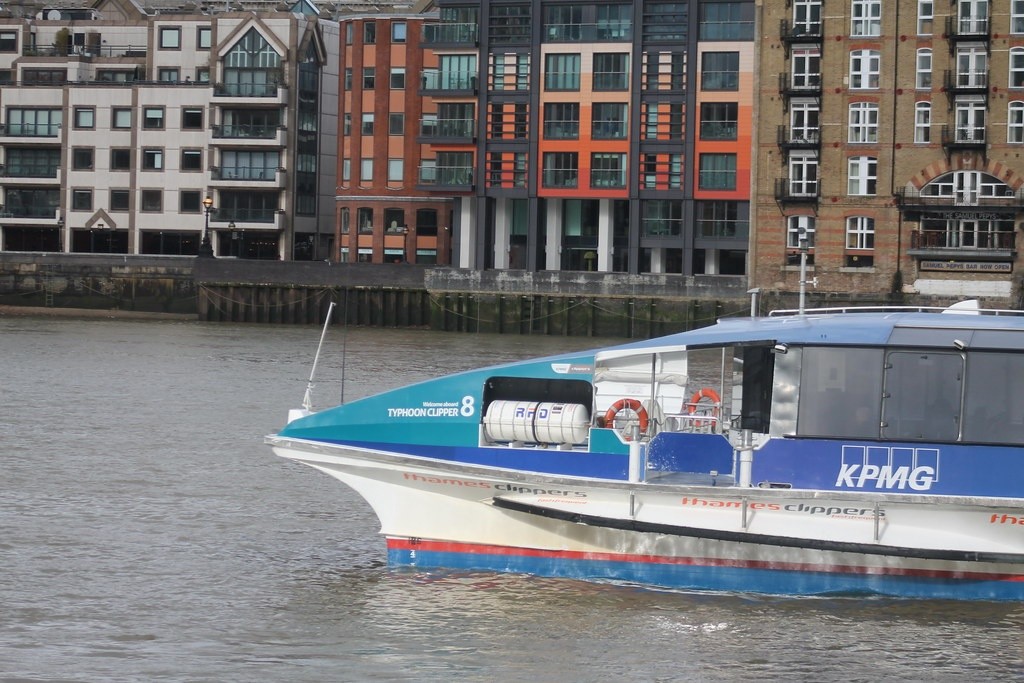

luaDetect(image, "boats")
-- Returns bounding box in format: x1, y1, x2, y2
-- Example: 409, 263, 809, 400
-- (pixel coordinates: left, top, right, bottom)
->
260, 294, 1022, 607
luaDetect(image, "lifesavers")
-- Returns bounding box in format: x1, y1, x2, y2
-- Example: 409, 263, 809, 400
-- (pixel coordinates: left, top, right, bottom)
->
688, 388, 722, 427
604, 398, 649, 441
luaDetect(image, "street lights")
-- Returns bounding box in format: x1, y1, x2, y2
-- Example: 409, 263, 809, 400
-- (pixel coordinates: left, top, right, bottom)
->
197, 195, 215, 258
798, 238, 808, 315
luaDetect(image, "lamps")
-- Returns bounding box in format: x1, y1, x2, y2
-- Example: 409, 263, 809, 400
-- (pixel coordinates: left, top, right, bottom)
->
953, 339, 969, 353
775, 342, 789, 354
